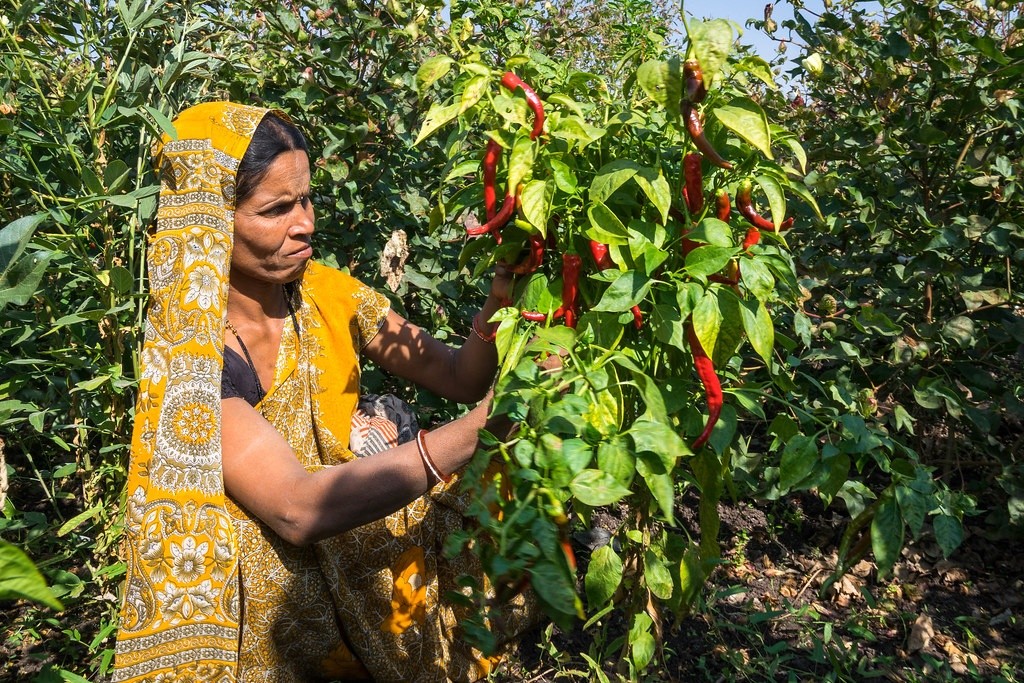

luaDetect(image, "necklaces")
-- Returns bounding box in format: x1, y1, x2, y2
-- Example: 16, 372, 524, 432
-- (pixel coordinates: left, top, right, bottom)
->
226, 300, 300, 425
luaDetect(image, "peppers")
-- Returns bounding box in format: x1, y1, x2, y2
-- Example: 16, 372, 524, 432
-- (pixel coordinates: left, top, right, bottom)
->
462, 45, 875, 621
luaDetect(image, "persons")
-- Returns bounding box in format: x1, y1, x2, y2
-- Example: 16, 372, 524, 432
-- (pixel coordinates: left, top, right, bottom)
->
108, 101, 571, 683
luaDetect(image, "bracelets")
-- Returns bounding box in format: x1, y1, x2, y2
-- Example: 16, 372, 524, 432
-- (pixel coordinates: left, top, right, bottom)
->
417, 429, 453, 482
472, 315, 497, 344
